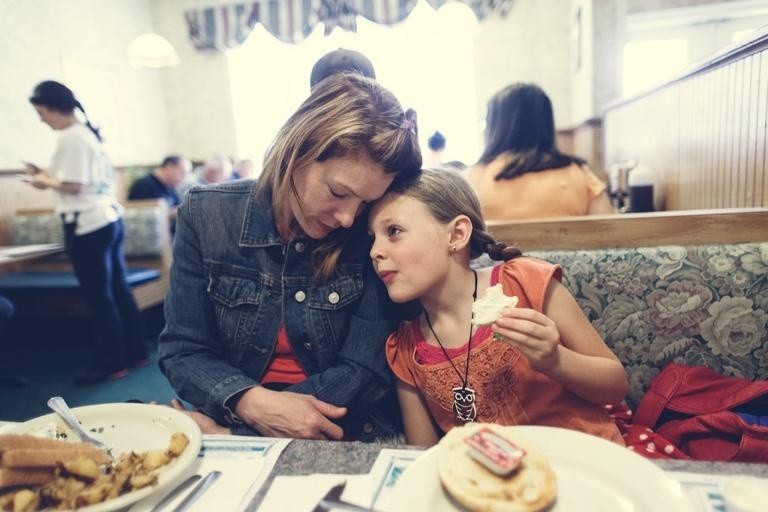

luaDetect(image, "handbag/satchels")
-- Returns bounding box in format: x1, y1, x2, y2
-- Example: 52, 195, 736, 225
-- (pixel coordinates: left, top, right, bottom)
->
631, 362, 767, 462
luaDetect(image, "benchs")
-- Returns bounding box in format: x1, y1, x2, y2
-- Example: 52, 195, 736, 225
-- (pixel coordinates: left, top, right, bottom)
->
418, 206, 768, 461
10, 197, 172, 332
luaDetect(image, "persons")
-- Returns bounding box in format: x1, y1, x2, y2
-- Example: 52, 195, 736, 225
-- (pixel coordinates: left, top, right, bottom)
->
22, 81, 147, 386
366, 169, 630, 454
310, 47, 376, 99
151, 74, 422, 444
422, 132, 457, 166
452, 84, 619, 221
129, 154, 192, 237
193, 146, 255, 189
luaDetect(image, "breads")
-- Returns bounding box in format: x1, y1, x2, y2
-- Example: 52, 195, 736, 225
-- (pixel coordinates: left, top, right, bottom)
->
0, 432, 191, 512
437, 420, 559, 512
471, 282, 520, 327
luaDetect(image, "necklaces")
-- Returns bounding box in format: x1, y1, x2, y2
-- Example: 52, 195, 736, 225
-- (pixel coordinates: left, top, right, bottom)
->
420, 267, 478, 425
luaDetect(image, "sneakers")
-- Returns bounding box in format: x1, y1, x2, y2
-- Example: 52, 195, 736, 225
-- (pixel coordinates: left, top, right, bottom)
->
70, 354, 150, 387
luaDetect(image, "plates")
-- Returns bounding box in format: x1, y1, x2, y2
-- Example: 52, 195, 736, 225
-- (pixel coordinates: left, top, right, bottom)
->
375, 425, 696, 511
22, 402, 202, 512
